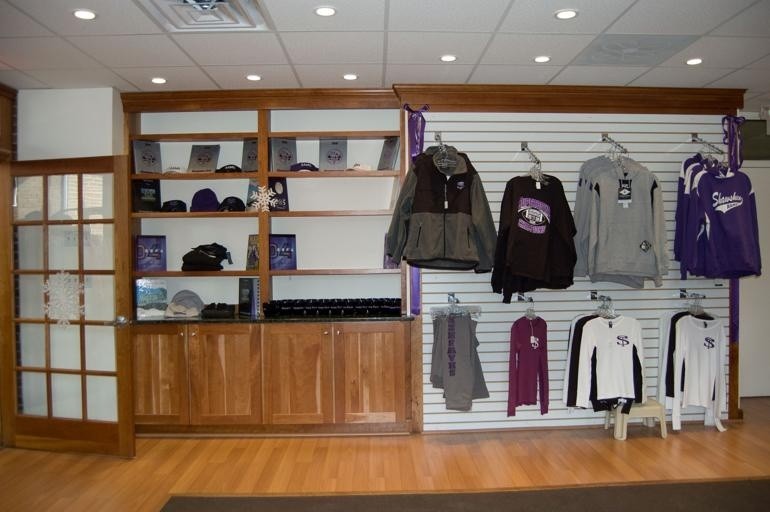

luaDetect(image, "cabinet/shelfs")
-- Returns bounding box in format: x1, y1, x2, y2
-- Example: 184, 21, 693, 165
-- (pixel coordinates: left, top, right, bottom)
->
120, 86, 425, 436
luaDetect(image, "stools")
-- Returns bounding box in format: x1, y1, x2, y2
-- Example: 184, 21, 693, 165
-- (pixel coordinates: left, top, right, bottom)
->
604, 397, 667, 441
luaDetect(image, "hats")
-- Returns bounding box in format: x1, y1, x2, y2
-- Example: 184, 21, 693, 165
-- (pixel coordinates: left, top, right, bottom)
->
159, 199, 187, 213
217, 196, 246, 212
190, 188, 220, 212
